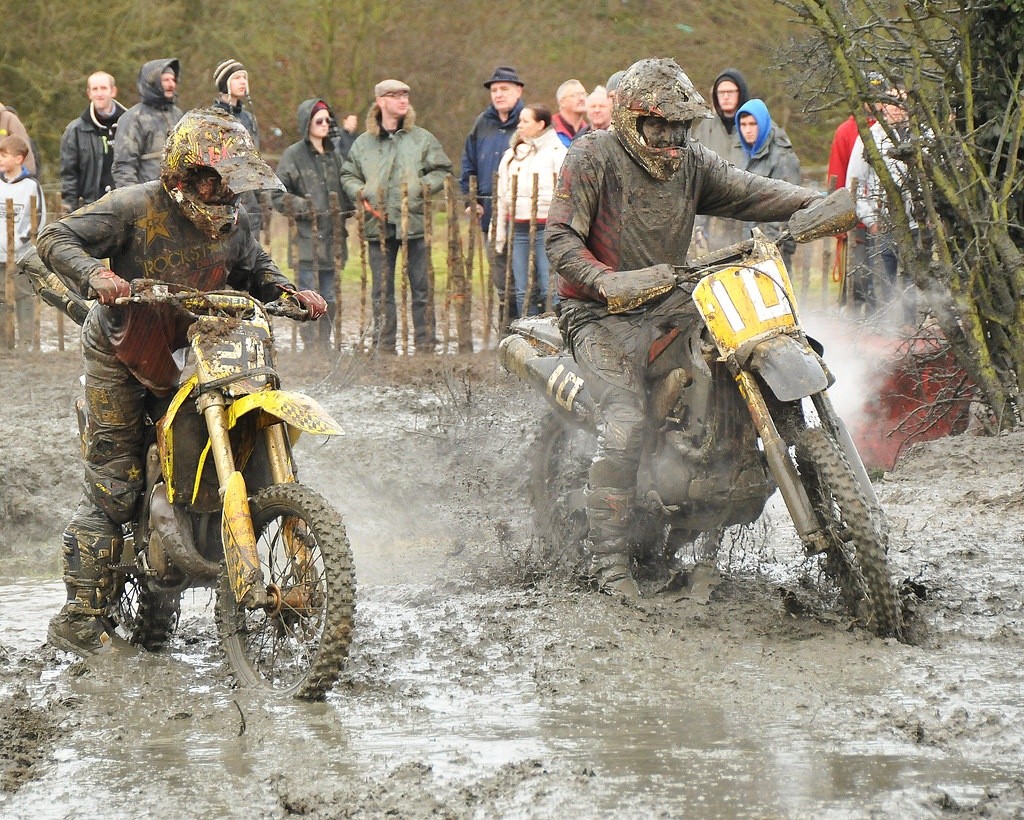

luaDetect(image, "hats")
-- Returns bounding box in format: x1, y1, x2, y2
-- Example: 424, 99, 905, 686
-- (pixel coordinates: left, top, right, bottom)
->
163, 66, 176, 75
212, 59, 258, 133
606, 70, 627, 97
483, 67, 524, 90
374, 80, 411, 97
311, 100, 329, 117
716, 77, 738, 88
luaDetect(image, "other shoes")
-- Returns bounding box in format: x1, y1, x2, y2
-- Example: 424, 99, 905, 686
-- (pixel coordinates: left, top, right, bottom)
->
49, 610, 139, 662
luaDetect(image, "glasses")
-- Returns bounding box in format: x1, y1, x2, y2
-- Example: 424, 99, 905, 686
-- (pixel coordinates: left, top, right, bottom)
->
312, 118, 332, 124
387, 92, 409, 98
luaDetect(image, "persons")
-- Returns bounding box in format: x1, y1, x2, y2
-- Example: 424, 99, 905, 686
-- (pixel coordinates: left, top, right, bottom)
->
111, 57, 185, 187
340, 113, 363, 162
692, 66, 802, 273
544, 56, 826, 602
459, 66, 527, 323
606, 70, 626, 102
845, 88, 945, 311
58, 70, 130, 214
551, 79, 588, 149
491, 102, 569, 318
272, 97, 355, 346
340, 78, 453, 351
0, 134, 47, 349
208, 57, 274, 245
828, 71, 892, 307
0, 102, 35, 178
35, 109, 329, 658
571, 86, 615, 141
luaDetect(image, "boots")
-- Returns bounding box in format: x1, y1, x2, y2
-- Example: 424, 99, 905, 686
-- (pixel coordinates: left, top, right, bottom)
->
581, 484, 638, 601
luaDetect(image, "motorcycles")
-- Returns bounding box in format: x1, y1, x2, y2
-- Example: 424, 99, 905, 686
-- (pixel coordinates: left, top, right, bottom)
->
496, 188, 914, 638
17, 244, 358, 707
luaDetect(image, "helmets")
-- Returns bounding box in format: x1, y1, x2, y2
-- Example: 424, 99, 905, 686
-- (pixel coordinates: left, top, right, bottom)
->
163, 108, 286, 240
611, 57, 713, 183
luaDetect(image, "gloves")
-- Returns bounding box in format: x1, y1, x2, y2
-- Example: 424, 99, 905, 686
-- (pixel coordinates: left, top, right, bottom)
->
88, 268, 131, 307
292, 290, 328, 321
